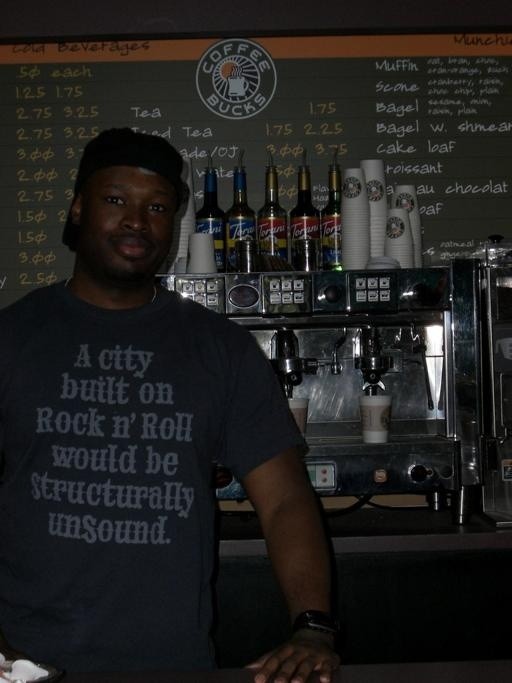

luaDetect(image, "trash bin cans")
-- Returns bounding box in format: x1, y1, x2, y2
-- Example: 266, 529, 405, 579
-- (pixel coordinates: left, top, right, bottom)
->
287, 610, 341, 638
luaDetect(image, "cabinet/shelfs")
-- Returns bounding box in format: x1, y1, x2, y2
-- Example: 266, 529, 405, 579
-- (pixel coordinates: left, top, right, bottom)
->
259, 163, 287, 266
291, 161, 320, 267
296, 239, 317, 271
196, 164, 226, 271
323, 161, 344, 269
227, 162, 257, 269
235, 241, 261, 271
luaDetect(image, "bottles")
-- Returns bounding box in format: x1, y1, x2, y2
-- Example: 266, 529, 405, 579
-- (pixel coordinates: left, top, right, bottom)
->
63, 128, 183, 246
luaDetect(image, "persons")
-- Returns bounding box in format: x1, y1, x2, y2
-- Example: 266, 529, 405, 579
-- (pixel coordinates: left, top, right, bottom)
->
1, 127, 341, 682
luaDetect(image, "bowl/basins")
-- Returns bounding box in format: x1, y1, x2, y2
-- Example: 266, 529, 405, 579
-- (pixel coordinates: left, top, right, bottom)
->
65, 273, 160, 306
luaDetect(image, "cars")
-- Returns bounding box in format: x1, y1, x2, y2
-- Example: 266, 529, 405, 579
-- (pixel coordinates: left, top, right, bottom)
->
359, 393, 393, 442
164, 155, 196, 261
342, 157, 422, 267
285, 396, 309, 436
186, 231, 220, 272
